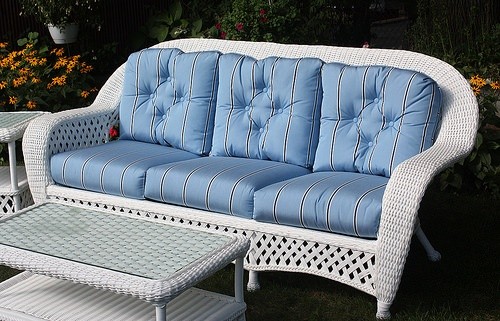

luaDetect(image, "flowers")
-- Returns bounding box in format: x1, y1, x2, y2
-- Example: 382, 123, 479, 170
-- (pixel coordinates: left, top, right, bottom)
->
18, 0, 104, 35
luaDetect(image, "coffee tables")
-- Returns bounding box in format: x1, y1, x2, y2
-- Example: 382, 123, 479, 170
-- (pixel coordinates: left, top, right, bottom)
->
0, 198, 251, 321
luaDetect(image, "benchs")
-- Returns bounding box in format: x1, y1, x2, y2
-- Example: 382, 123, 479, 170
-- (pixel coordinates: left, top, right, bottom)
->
20, 37, 481, 320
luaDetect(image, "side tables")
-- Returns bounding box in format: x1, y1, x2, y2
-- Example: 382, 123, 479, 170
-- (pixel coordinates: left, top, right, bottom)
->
0, 110, 53, 220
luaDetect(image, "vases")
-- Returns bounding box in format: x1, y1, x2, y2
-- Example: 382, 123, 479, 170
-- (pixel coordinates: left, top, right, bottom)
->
44, 21, 82, 45
